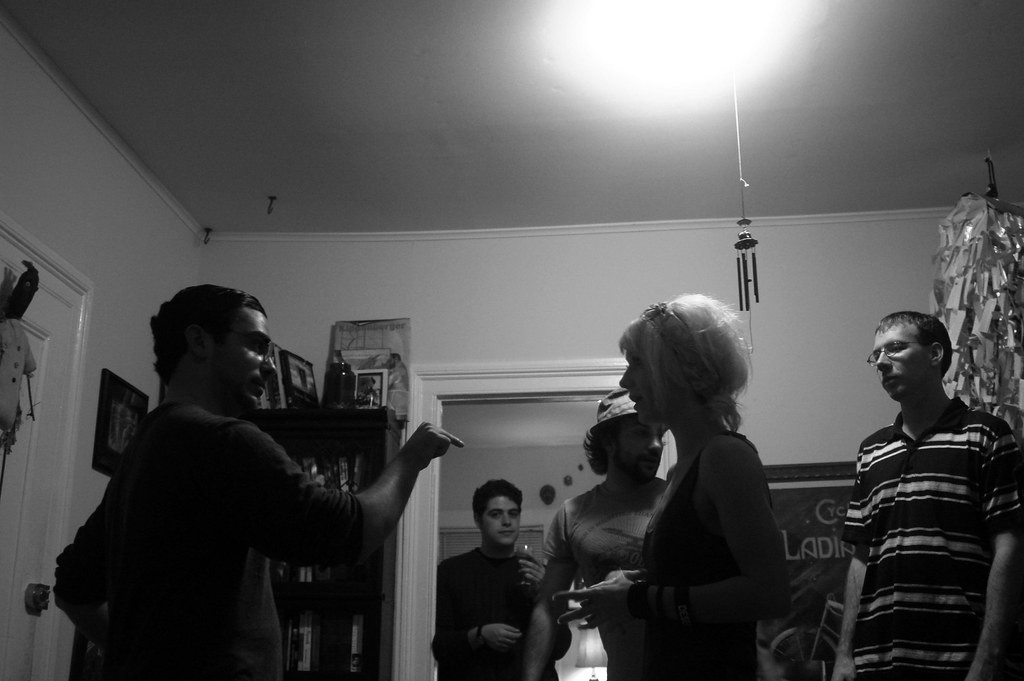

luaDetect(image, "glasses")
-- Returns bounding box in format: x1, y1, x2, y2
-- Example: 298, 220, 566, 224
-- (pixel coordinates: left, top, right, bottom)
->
867, 341, 920, 367
231, 327, 283, 366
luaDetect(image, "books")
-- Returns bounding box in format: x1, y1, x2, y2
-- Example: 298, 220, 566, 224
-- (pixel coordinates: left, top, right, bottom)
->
270, 561, 363, 676
292, 451, 368, 495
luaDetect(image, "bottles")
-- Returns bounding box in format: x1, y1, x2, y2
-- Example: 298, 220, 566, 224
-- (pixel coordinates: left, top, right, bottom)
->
323, 363, 356, 407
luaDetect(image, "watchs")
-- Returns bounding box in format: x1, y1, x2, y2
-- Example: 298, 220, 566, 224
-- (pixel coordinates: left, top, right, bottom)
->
476, 623, 487, 648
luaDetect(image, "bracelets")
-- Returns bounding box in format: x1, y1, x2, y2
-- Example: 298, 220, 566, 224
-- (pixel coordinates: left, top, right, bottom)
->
625, 579, 694, 626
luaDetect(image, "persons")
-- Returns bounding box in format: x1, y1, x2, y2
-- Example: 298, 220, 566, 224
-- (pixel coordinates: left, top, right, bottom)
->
553, 296, 789, 681
523, 388, 670, 681
831, 311, 1024, 681
431, 478, 571, 681
50, 285, 463, 681
383, 351, 410, 422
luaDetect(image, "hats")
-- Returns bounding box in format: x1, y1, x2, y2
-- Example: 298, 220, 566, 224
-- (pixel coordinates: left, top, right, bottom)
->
590, 388, 667, 436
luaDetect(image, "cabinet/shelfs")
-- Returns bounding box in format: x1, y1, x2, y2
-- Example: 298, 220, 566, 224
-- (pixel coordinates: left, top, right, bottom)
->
70, 407, 403, 681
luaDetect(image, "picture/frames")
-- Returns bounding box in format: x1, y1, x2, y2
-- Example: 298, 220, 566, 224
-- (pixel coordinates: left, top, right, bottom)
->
354, 369, 388, 409
92, 368, 149, 477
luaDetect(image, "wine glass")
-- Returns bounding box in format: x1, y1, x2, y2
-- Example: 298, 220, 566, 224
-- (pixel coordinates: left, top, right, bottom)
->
515, 544, 534, 585
